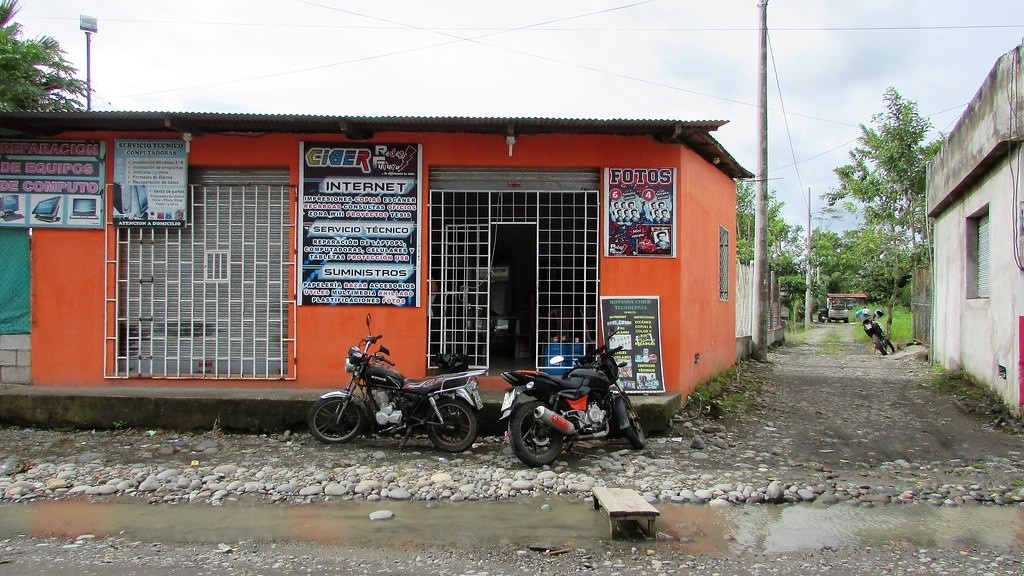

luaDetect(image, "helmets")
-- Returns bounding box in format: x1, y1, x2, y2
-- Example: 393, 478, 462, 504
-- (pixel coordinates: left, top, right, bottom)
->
875, 309, 884, 318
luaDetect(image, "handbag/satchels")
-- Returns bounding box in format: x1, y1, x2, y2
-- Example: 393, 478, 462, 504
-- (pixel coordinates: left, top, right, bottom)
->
436, 352, 470, 376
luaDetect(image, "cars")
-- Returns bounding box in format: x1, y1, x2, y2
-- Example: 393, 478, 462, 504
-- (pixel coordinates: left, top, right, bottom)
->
818, 308, 828, 322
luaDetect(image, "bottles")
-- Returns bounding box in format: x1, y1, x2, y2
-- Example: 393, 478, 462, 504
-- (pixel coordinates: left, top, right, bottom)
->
544, 336, 584, 374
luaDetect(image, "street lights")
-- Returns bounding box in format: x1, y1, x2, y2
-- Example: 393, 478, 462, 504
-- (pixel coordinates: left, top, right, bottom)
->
80, 15, 97, 113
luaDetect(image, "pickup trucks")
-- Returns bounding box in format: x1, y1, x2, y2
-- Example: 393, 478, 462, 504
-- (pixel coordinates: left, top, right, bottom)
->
828, 302, 849, 323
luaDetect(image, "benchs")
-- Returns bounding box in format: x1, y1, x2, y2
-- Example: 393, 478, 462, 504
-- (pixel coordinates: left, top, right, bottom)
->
593, 486, 661, 540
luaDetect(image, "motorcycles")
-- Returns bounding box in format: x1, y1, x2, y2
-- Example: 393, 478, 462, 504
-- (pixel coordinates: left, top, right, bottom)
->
857, 309, 896, 356
501, 328, 646, 467
307, 312, 484, 454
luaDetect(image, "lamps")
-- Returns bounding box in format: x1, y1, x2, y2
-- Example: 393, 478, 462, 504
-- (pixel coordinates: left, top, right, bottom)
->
505, 136, 516, 156
183, 133, 192, 154
